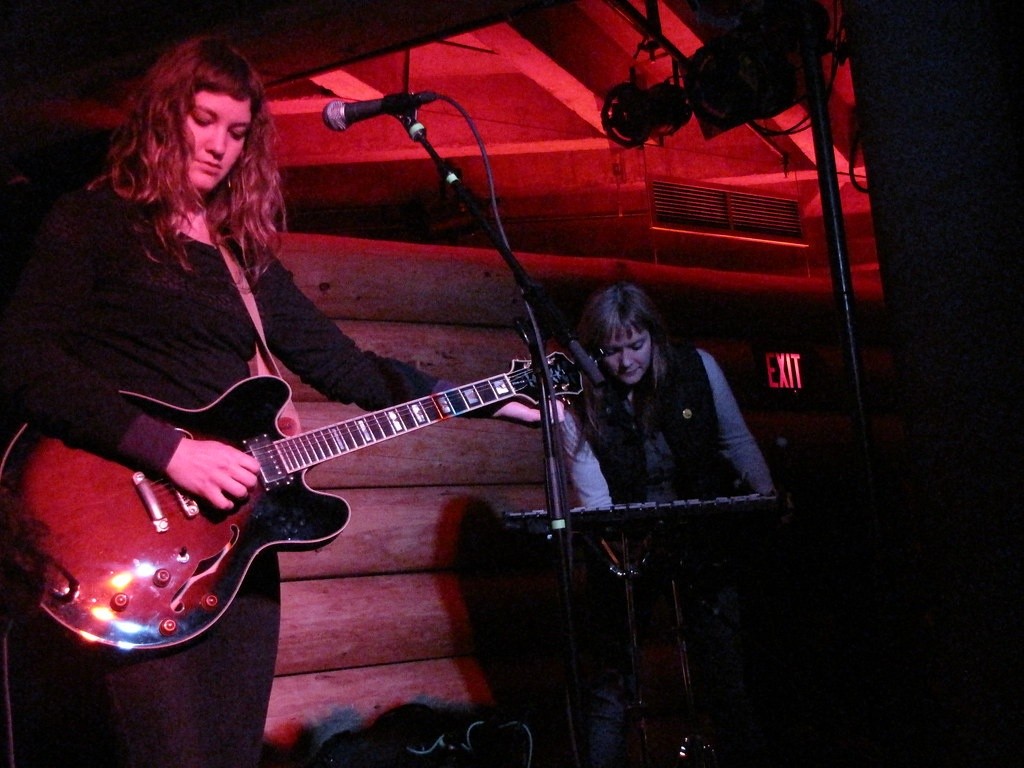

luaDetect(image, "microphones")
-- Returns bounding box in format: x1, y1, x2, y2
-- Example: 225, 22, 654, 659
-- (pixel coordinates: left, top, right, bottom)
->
322, 90, 436, 131
603, 80, 692, 146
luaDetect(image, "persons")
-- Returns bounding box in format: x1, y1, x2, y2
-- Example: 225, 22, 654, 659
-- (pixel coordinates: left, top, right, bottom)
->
552, 283, 791, 768
0, 36, 562, 768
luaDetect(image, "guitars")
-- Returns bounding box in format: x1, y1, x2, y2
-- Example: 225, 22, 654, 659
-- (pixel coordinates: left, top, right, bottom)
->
0, 352, 583, 658
